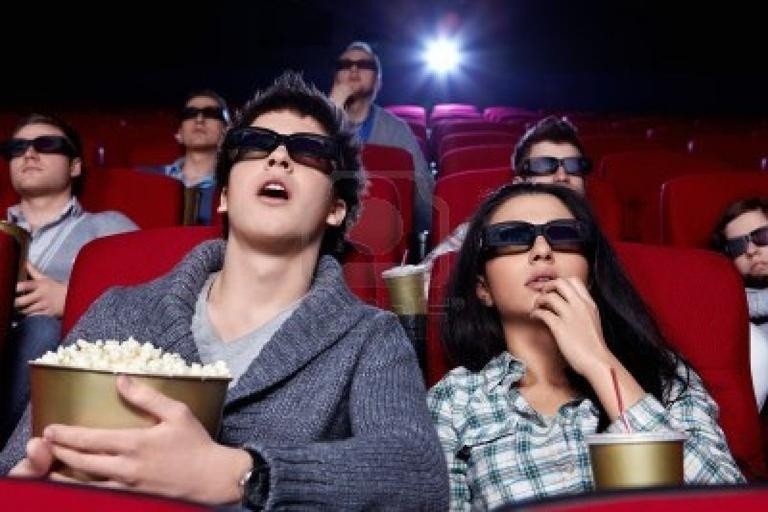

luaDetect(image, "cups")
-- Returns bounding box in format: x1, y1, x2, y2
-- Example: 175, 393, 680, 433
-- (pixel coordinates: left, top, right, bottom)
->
1, 220, 33, 293
381, 272, 431, 317
26, 357, 235, 486
581, 427, 687, 488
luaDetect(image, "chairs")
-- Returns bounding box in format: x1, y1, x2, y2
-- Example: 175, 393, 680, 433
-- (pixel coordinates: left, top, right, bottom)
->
2, 110, 222, 338
1, 476, 201, 509
343, 103, 766, 510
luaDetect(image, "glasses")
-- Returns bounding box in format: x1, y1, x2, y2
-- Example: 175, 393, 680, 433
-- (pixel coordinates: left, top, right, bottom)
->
476, 218, 594, 254
719, 226, 768, 259
181, 106, 230, 126
518, 157, 593, 175
0, 136, 76, 158
220, 126, 343, 175
334, 58, 375, 70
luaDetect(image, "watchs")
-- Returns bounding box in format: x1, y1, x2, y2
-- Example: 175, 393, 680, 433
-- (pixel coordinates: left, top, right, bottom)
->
240, 446, 269, 510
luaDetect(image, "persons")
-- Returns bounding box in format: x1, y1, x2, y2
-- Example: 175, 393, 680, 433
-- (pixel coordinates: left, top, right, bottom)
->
2, 68, 450, 511
427, 180, 748, 512
1, 114, 142, 448
327, 42, 436, 234
160, 90, 231, 224
417, 115, 586, 301
706, 194, 768, 415
